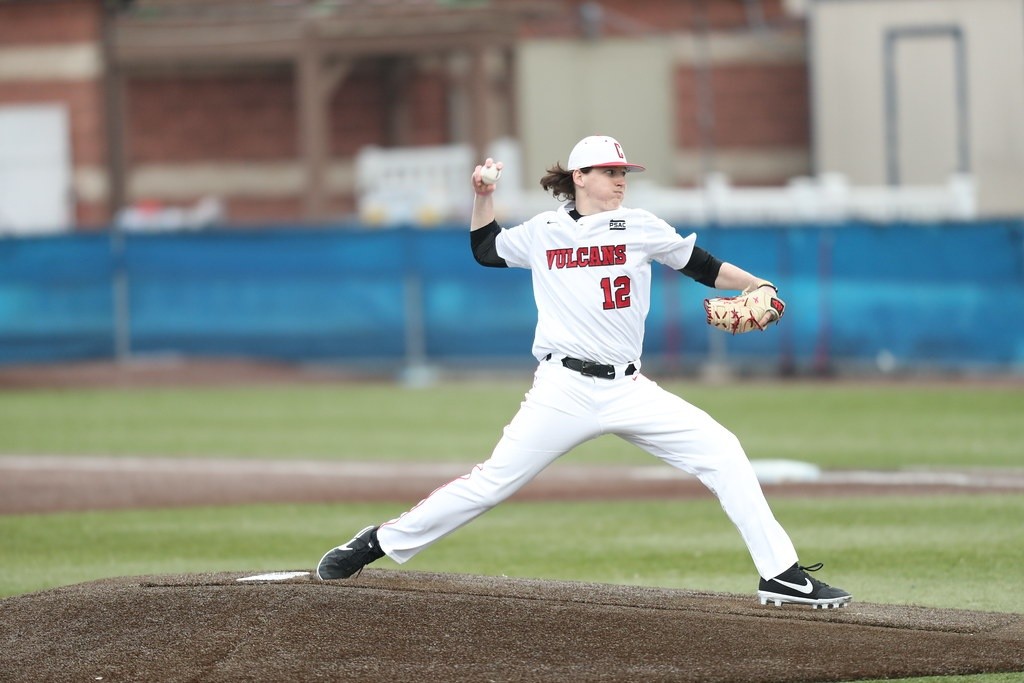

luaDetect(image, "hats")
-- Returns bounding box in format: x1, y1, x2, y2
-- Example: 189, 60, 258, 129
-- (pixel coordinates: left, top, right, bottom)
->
567, 133, 647, 174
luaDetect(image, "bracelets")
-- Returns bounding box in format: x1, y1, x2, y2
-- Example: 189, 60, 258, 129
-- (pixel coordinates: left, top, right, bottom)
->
755, 280, 777, 293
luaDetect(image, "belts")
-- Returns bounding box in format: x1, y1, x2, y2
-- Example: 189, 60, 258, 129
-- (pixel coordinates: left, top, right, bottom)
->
546, 353, 637, 380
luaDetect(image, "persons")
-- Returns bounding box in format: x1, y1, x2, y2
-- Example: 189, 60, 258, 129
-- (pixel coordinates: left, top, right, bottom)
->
316, 136, 853, 612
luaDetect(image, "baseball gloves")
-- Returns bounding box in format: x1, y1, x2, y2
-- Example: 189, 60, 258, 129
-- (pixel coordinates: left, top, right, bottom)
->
704, 285, 786, 336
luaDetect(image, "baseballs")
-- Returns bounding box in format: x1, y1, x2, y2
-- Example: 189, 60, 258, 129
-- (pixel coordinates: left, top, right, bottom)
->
480, 163, 503, 186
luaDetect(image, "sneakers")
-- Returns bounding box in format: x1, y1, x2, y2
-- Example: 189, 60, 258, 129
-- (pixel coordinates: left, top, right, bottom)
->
758, 561, 852, 610
317, 526, 385, 581
